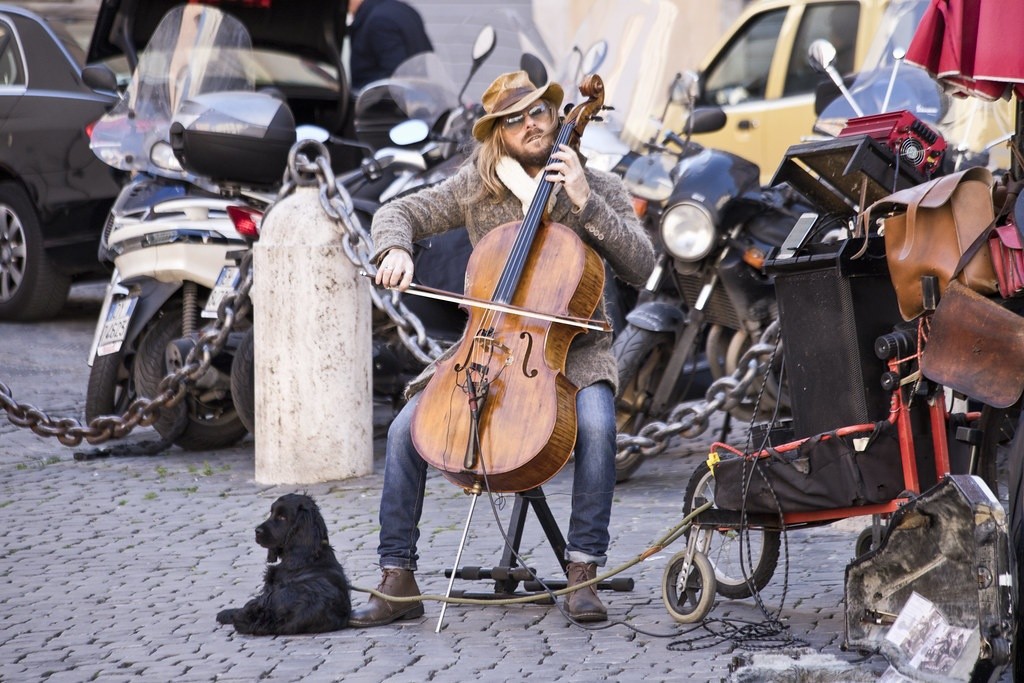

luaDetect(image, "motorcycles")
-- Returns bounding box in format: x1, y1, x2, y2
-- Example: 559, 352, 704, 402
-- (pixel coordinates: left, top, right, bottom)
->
85, 4, 1020, 488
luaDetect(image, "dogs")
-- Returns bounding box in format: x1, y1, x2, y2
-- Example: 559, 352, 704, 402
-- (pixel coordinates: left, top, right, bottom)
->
215, 490, 351, 636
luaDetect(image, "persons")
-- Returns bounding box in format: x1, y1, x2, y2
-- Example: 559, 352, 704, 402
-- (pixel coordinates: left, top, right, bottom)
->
350, 70, 656, 629
344, 0, 434, 168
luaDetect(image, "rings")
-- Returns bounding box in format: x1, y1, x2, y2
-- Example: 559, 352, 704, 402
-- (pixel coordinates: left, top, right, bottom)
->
385, 266, 393, 271
405, 271, 414, 276
560, 177, 566, 184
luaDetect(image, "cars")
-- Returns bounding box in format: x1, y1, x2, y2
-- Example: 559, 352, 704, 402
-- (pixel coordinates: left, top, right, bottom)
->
0, 6, 131, 324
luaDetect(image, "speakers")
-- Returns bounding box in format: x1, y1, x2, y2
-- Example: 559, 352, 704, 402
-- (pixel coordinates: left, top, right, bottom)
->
772, 264, 918, 440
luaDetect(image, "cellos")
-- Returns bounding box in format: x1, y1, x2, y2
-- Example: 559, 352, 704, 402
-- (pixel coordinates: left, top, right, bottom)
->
409, 70, 607, 632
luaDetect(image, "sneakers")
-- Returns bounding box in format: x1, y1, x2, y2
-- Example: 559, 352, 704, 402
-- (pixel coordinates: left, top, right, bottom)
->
562, 562, 607, 620
348, 563, 425, 626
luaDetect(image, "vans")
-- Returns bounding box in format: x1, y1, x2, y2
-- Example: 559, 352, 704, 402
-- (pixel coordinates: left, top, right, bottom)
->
659, 1, 887, 178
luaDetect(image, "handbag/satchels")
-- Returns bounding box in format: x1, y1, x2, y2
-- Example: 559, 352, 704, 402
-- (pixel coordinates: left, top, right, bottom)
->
847, 167, 1024, 408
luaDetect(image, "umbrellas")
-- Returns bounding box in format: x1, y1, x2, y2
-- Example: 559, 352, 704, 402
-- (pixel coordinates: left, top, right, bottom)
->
903, 1, 1024, 182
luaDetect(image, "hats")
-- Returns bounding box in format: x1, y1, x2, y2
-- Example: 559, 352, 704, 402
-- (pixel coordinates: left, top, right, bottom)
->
471, 71, 564, 143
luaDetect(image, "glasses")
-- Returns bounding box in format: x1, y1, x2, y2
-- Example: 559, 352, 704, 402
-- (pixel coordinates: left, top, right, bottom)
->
501, 103, 550, 130
